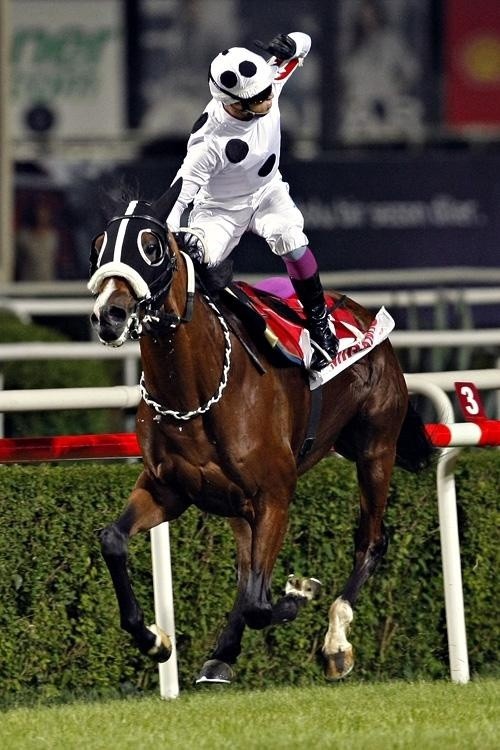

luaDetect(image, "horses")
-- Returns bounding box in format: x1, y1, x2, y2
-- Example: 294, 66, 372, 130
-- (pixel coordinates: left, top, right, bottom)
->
87, 176, 449, 685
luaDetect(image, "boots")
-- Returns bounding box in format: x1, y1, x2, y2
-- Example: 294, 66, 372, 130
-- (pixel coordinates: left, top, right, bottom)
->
290, 269, 338, 369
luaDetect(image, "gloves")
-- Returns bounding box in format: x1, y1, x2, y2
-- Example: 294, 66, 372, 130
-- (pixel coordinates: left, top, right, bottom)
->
252, 33, 296, 59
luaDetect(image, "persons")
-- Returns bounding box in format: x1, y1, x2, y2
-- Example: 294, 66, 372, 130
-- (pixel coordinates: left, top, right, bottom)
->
166, 31, 340, 369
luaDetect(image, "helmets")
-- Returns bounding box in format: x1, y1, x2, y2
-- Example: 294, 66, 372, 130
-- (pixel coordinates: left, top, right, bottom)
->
208, 47, 273, 105
